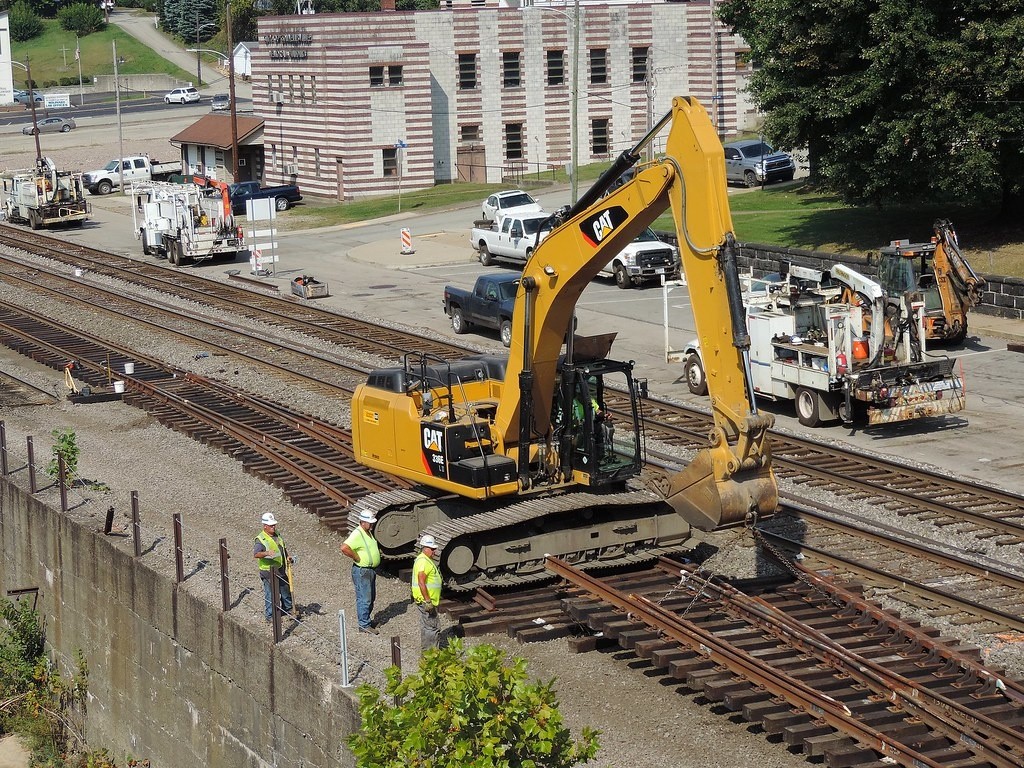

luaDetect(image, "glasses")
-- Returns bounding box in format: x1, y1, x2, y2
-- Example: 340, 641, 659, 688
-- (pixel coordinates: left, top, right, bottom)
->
428, 547, 436, 551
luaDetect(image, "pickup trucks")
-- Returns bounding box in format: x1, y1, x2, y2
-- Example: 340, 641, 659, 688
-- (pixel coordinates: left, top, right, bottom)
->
82, 154, 183, 194
595, 225, 680, 290
441, 271, 578, 348
469, 213, 556, 267
208, 180, 303, 213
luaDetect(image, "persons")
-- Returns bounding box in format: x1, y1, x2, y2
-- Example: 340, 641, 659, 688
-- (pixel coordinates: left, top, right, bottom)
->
556, 382, 613, 427
340, 509, 381, 635
253, 513, 294, 623
412, 535, 443, 660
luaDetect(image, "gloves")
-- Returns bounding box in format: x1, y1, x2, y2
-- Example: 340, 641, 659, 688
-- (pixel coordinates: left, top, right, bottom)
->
424, 602, 438, 617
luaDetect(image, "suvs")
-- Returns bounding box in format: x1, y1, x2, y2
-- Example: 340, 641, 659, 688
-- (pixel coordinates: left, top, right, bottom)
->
164, 88, 201, 104
210, 93, 232, 111
721, 139, 796, 186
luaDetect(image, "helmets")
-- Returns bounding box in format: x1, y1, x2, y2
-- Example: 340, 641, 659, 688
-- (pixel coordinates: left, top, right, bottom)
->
262, 513, 277, 525
420, 535, 438, 548
358, 509, 378, 524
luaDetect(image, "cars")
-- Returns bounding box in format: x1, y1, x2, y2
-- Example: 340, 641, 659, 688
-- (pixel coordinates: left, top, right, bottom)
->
14, 90, 43, 103
23, 116, 77, 135
481, 189, 545, 221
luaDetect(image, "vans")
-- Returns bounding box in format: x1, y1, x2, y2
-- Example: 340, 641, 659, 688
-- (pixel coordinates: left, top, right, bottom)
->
598, 167, 643, 198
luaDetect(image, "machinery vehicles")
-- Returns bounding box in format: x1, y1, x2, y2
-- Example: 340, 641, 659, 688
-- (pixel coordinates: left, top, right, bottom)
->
682, 266, 966, 431
5, 156, 95, 231
129, 175, 248, 267
843, 217, 985, 346
344, 96, 783, 593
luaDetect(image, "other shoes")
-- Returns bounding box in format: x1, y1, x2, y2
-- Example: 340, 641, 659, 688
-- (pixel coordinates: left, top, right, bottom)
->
269, 617, 274, 623
359, 624, 379, 635
287, 609, 294, 615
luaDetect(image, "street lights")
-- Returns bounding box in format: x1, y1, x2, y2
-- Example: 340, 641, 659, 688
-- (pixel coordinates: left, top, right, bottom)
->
197, 22, 215, 87
184, 48, 240, 185
